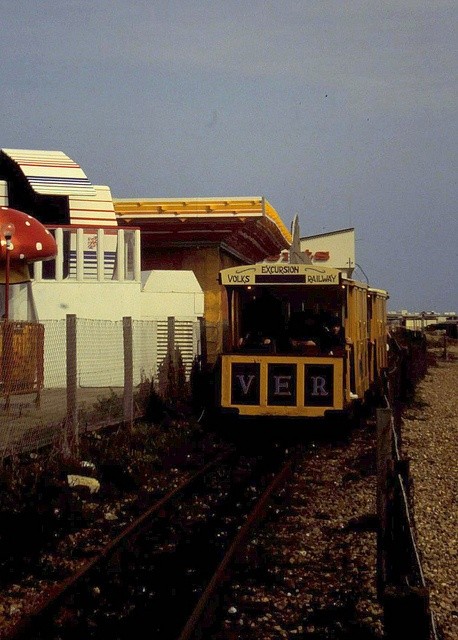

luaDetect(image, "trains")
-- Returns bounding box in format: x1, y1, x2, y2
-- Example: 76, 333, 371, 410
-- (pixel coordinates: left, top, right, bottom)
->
216, 263, 388, 416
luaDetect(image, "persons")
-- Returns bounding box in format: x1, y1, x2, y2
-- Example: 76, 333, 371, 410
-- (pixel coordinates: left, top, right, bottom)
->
324, 322, 346, 356
